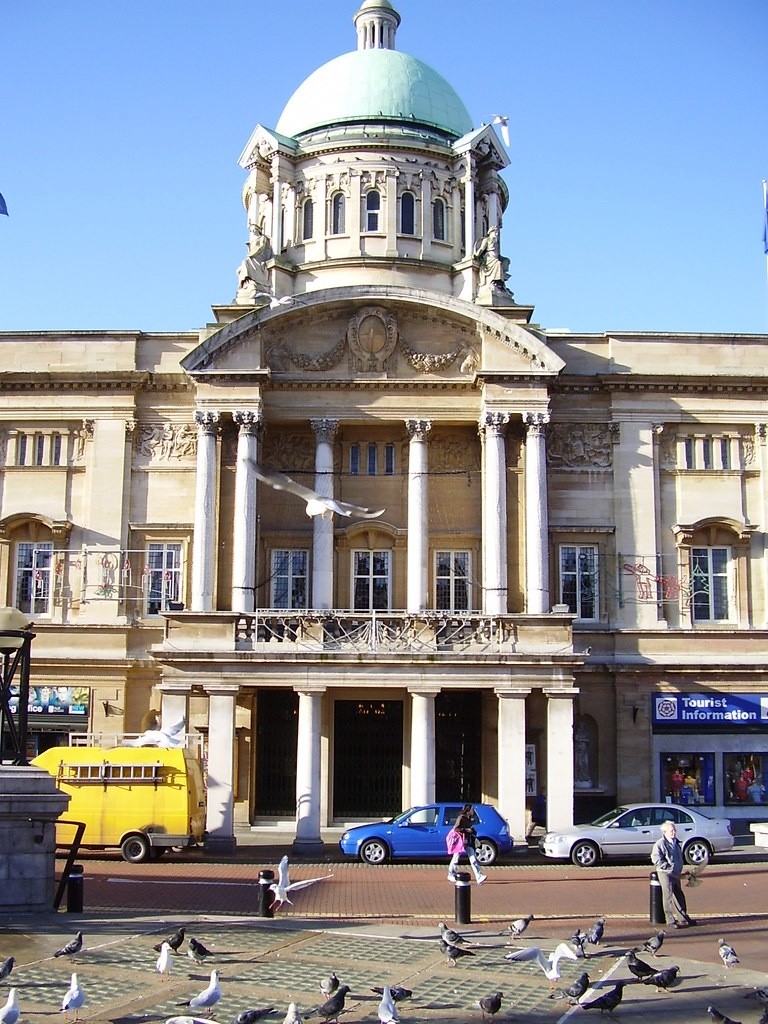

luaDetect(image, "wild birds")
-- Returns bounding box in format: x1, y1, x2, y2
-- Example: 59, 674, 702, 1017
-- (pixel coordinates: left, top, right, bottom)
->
165, 1016, 221, 1024
624, 951, 658, 983
717, 938, 740, 968
581, 981, 627, 1018
240, 456, 386, 521
302, 973, 351, 1024
0, 988, 23, 1024
0, 956, 15, 981
438, 914, 606, 1007
232, 1007, 279, 1024
643, 966, 681, 993
152, 928, 214, 981
176, 969, 223, 1016
371, 986, 413, 1005
378, 986, 400, 1024
266, 855, 334, 911
478, 992, 505, 1023
54, 932, 82, 963
643, 929, 666, 957
283, 1002, 303, 1024
59, 972, 85, 1023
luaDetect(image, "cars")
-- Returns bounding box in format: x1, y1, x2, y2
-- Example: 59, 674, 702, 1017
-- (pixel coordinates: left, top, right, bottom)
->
538, 803, 736, 866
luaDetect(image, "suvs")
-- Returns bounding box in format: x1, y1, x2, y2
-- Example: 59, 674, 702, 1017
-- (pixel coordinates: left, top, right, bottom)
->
339, 801, 515, 866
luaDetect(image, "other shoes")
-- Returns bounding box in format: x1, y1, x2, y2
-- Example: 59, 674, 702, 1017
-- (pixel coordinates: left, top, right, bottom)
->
478, 876, 486, 884
448, 875, 456, 882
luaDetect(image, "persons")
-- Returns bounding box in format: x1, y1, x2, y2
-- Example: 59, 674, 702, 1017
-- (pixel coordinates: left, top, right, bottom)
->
142, 423, 195, 459
446, 804, 487, 885
574, 724, 592, 781
9, 687, 72, 708
634, 812, 645, 825
728, 763, 764, 803
546, 423, 613, 467
672, 767, 701, 801
651, 820, 697, 928
477, 226, 511, 289
237, 224, 271, 289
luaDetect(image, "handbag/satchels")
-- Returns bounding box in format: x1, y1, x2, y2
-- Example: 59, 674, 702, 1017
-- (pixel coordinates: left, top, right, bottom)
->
474, 838, 481, 848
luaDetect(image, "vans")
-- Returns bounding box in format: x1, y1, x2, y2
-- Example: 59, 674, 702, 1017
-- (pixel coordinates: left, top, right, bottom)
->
26, 732, 206, 863
